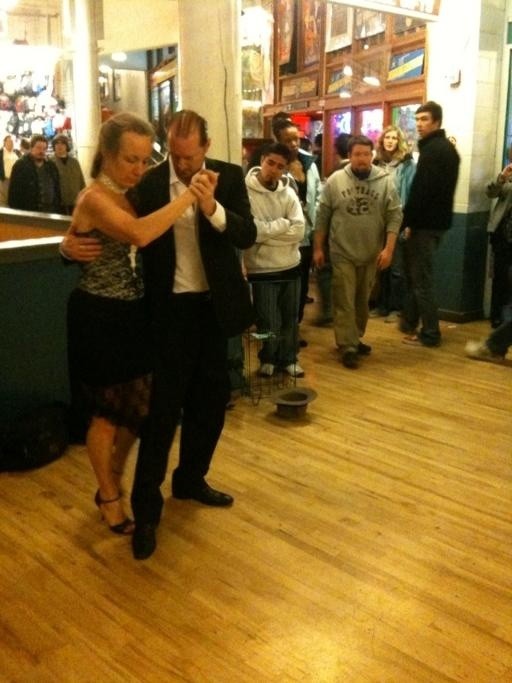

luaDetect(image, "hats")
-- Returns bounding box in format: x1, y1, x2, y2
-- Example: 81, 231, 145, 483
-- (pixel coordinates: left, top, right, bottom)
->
52, 134, 72, 151
269, 386, 317, 418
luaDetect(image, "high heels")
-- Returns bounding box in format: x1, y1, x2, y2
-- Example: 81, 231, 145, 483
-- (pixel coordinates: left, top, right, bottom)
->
95, 484, 136, 534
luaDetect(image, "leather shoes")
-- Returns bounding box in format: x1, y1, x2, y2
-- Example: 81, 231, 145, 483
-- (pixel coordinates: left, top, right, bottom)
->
173, 477, 233, 505
133, 511, 161, 559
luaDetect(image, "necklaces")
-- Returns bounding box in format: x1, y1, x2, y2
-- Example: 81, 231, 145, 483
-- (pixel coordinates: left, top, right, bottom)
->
96, 172, 127, 196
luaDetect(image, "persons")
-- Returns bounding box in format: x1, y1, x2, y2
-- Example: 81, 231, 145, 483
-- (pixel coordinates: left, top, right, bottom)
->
464, 320, 511, 363
59, 110, 256, 561
313, 137, 402, 365
70, 117, 221, 533
52, 135, 84, 214
485, 165, 511, 328
8, 138, 60, 213
1, 138, 21, 208
311, 132, 354, 325
239, 110, 324, 379
399, 101, 460, 347
372, 126, 417, 323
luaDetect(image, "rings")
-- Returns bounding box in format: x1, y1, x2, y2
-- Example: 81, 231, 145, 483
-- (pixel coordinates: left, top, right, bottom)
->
78, 243, 88, 253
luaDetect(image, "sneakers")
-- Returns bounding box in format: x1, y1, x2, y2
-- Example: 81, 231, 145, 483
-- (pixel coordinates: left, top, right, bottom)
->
256, 293, 505, 377
